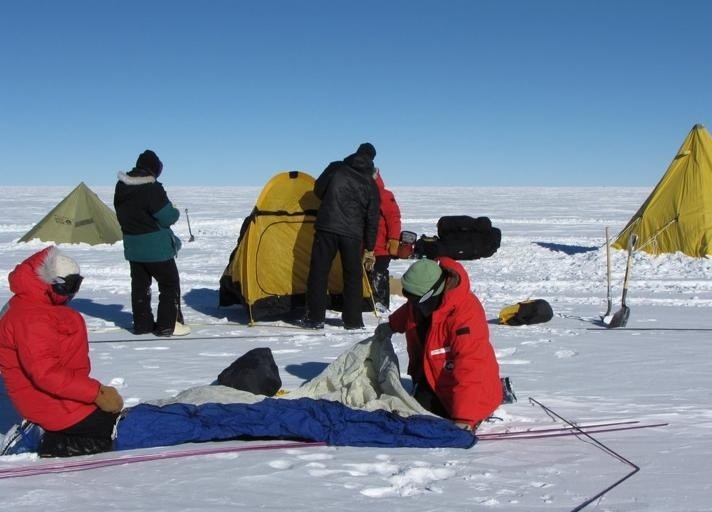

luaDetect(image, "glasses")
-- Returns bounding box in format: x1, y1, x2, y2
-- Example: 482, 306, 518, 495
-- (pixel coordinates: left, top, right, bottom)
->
402, 271, 445, 304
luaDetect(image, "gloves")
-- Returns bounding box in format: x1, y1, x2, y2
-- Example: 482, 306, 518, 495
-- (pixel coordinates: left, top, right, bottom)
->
94, 384, 124, 414
386, 239, 400, 256
363, 249, 376, 271
374, 322, 393, 339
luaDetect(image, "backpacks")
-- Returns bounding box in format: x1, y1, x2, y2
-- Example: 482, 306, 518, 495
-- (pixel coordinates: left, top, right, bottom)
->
498, 300, 554, 325
398, 215, 502, 260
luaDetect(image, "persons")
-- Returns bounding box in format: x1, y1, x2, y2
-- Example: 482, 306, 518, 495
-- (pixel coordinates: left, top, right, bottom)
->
369, 169, 402, 313
374, 256, 504, 436
114, 150, 181, 338
292, 142, 378, 331
0, 246, 123, 458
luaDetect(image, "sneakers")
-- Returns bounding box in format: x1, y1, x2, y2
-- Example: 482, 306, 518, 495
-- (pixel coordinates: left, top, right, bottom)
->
1, 417, 45, 456
499, 377, 517, 402
344, 321, 365, 329
134, 314, 190, 337
376, 302, 390, 313
301, 317, 324, 329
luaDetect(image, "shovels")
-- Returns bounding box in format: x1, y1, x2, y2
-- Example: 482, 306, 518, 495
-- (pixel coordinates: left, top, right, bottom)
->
185, 208, 194, 242
601, 226, 638, 327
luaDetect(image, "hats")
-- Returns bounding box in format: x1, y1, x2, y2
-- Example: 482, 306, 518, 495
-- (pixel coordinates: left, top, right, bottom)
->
401, 259, 446, 297
356, 143, 377, 160
49, 255, 81, 284
136, 149, 163, 176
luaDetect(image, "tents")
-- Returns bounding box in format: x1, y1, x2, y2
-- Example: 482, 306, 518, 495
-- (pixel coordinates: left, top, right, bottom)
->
16, 182, 125, 247
217, 170, 378, 325
603, 123, 712, 258
